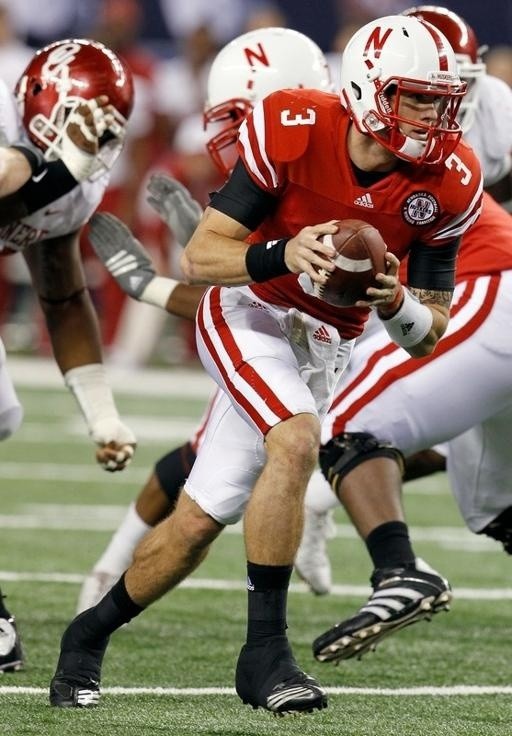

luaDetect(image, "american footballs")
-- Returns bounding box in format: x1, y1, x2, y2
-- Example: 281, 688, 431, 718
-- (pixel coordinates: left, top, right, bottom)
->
313, 218, 389, 307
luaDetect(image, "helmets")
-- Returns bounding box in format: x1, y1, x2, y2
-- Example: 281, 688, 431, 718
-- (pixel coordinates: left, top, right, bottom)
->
340, 4, 487, 169
13, 39, 135, 183
202, 27, 330, 181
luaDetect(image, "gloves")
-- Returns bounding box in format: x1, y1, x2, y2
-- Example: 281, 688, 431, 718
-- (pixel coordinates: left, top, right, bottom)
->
57, 94, 115, 182
88, 210, 156, 300
147, 173, 202, 247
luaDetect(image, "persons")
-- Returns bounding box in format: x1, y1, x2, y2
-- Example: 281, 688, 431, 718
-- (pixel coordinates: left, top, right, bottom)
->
47, 14, 486, 718
64, 2, 512, 613
0, 1, 509, 365
1, 41, 137, 679
84, 24, 511, 669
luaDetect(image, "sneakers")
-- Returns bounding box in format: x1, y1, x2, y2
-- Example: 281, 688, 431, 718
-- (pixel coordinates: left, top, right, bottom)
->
236, 645, 328, 718
313, 557, 454, 666
0, 591, 22, 674
50, 615, 109, 709
293, 503, 338, 596
